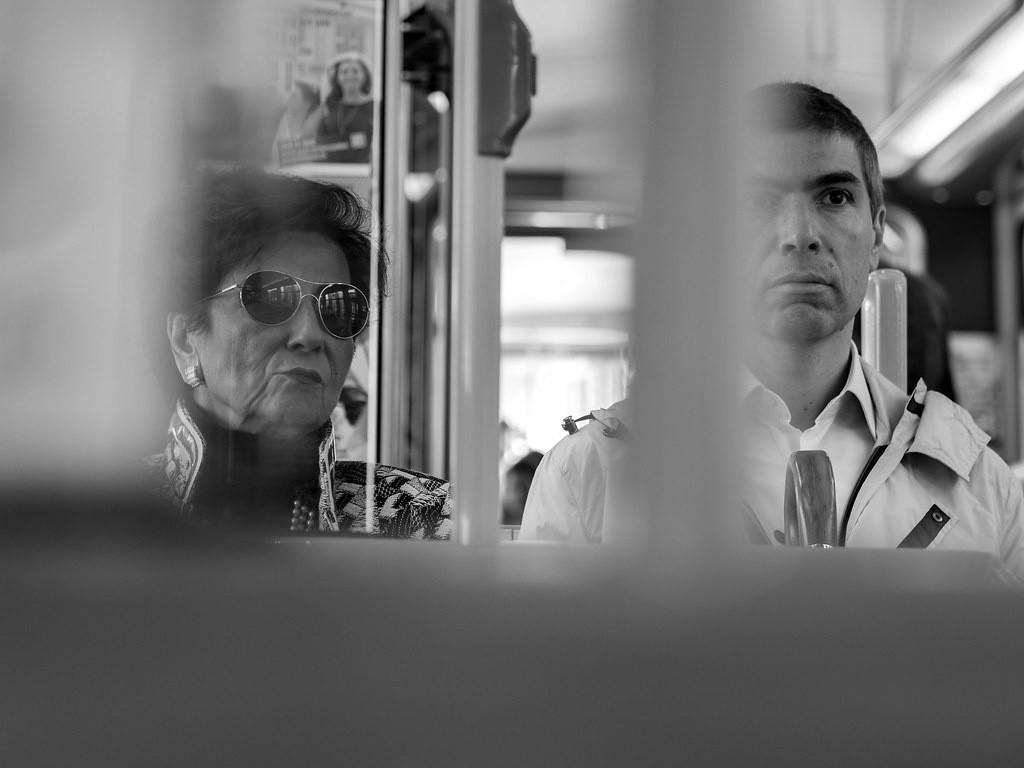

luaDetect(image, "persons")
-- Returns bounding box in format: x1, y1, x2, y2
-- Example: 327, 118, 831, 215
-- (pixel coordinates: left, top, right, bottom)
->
521, 82, 1023, 582
140, 168, 453, 539
275, 56, 373, 164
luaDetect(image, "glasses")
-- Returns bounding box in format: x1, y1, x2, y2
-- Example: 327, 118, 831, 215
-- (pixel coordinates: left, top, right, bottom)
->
182, 268, 369, 339
